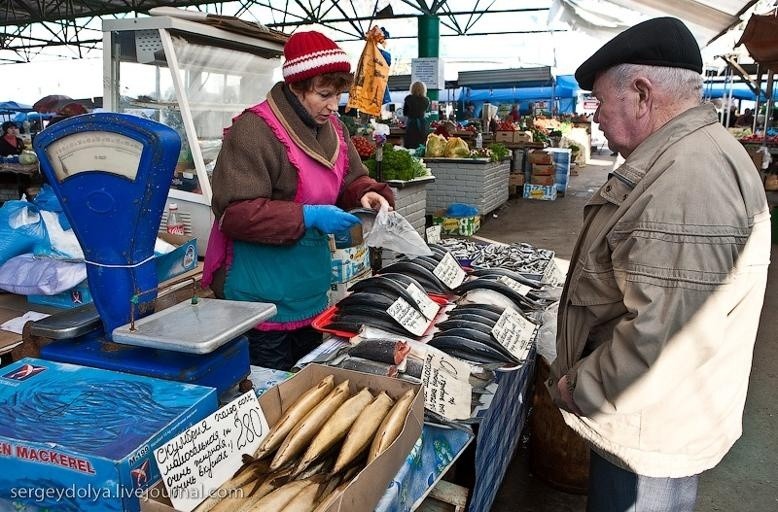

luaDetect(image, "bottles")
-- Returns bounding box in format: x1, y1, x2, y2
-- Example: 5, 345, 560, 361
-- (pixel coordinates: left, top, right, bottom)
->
165, 203, 185, 236
476, 133, 483, 149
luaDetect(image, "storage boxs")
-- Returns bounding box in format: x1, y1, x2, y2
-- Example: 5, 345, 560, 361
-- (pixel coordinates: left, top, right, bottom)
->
0, 356, 425, 512
432, 215, 480, 237
509, 151, 558, 201
154, 231, 198, 285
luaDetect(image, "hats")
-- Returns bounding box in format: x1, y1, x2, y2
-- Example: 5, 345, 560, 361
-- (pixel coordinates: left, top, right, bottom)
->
574, 17, 703, 91
283, 30, 351, 83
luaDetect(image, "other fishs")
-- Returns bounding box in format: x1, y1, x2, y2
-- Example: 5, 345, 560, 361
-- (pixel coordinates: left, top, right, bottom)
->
320, 237, 555, 438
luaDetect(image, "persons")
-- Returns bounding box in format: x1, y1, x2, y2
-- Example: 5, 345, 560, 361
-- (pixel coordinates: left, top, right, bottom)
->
734, 108, 754, 128
467, 102, 477, 118
201, 30, 395, 372
0, 122, 24, 157
544, 16, 772, 512
403, 80, 430, 149
510, 103, 521, 120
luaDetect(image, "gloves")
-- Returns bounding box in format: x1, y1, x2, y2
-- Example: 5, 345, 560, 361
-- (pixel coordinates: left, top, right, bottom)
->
303, 204, 358, 235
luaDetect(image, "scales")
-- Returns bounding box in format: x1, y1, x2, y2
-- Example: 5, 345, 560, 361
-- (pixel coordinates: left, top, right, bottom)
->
29, 112, 278, 398
